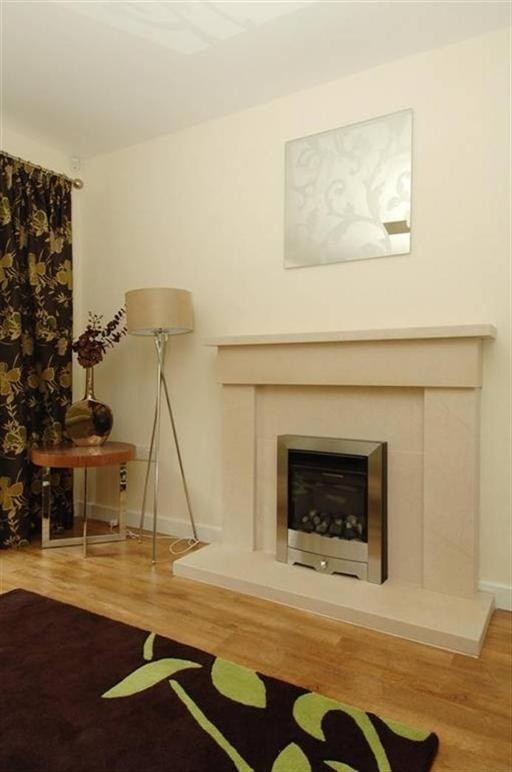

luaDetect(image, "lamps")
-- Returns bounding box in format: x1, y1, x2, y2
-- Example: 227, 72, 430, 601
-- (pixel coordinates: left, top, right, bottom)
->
123, 286, 198, 566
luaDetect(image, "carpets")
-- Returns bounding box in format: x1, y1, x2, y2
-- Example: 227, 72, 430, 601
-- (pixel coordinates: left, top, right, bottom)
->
1, 586, 439, 772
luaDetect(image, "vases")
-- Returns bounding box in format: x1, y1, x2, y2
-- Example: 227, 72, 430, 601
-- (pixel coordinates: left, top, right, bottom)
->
65, 366, 112, 447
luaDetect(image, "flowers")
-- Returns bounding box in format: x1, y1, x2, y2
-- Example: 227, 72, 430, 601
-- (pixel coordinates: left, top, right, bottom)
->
71, 303, 128, 367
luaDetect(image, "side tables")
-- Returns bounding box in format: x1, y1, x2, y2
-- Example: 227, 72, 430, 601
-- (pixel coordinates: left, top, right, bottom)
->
31, 440, 135, 560
